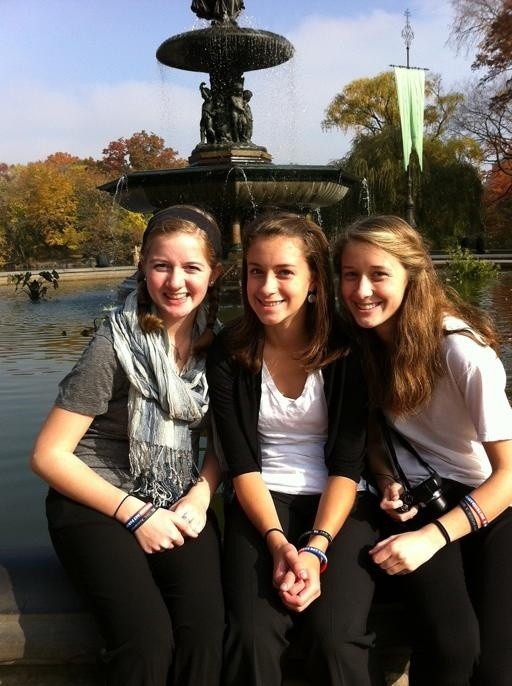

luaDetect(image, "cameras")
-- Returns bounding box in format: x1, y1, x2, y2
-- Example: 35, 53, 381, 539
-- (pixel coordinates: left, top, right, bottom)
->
395, 472, 451, 516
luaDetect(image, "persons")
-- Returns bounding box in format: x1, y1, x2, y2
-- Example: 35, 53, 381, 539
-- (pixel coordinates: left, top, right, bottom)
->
199, 81, 253, 144
334, 214, 512, 685
205, 208, 388, 685
30, 202, 229, 684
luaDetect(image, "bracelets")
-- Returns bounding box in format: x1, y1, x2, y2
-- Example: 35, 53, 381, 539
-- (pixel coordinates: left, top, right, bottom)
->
298, 547, 328, 574
129, 505, 158, 534
463, 495, 488, 528
458, 499, 479, 532
432, 520, 452, 546
113, 494, 130, 517
264, 528, 288, 547
125, 502, 152, 530
298, 529, 333, 547
